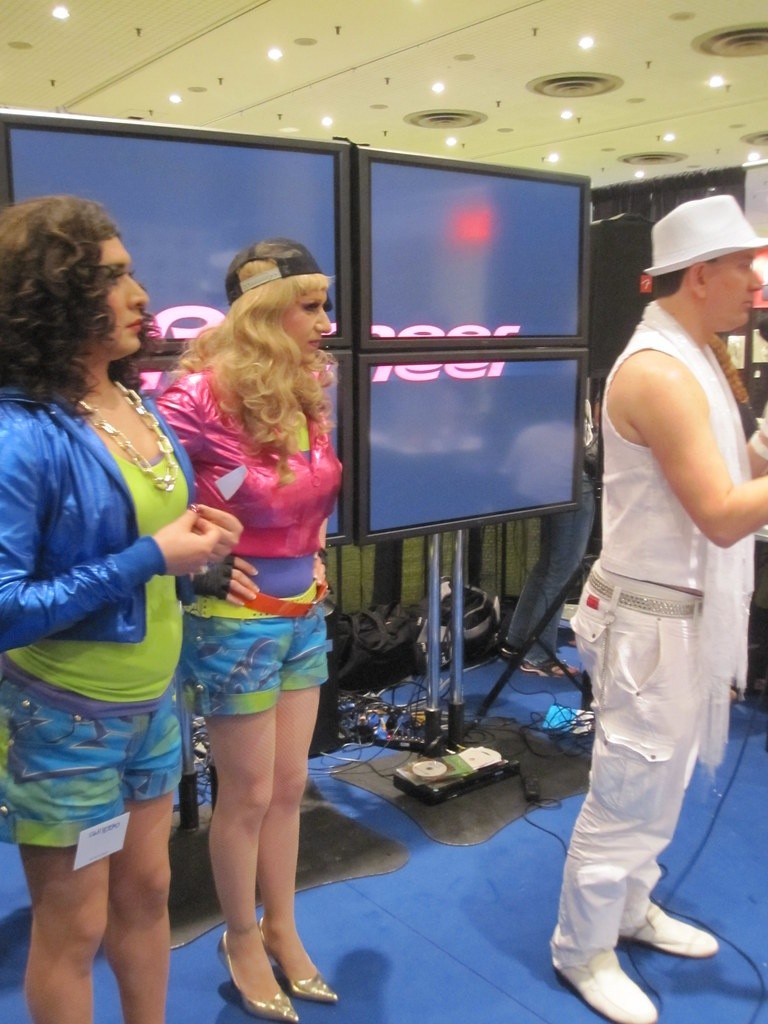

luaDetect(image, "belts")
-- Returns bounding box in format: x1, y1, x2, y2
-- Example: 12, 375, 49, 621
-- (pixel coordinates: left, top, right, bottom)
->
588, 569, 703, 619
228, 585, 326, 616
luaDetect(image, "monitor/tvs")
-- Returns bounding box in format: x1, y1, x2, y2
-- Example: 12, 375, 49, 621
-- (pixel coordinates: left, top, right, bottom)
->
0, 109, 590, 551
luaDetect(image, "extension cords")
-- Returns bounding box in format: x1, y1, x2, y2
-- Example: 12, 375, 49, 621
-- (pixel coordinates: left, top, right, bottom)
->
374, 734, 426, 753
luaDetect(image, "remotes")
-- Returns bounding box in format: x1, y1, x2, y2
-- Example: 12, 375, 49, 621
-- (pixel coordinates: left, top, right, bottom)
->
520, 770, 540, 802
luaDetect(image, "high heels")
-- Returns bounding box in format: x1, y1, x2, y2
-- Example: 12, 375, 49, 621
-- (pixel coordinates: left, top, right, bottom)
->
216, 918, 339, 1022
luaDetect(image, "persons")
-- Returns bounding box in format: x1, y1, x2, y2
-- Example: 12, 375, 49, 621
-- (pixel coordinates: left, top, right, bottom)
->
158, 240, 343, 1021
550, 196, 768, 1023
495, 393, 595, 677
0, 195, 244, 1024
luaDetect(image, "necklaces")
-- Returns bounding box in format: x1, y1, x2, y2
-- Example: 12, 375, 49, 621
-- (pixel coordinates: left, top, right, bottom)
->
73, 382, 177, 494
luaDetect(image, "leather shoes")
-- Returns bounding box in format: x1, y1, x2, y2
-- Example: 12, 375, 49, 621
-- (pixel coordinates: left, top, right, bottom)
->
554, 951, 659, 1024
619, 904, 719, 959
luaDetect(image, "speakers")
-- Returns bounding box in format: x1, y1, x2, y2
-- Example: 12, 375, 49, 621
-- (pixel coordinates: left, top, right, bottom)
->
589, 213, 678, 380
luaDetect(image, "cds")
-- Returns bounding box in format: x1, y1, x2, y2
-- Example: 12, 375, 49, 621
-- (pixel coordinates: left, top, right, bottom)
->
412, 760, 447, 777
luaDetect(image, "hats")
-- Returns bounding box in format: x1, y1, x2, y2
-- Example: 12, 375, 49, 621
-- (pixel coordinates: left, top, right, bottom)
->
226, 239, 334, 312
645, 195, 768, 276
